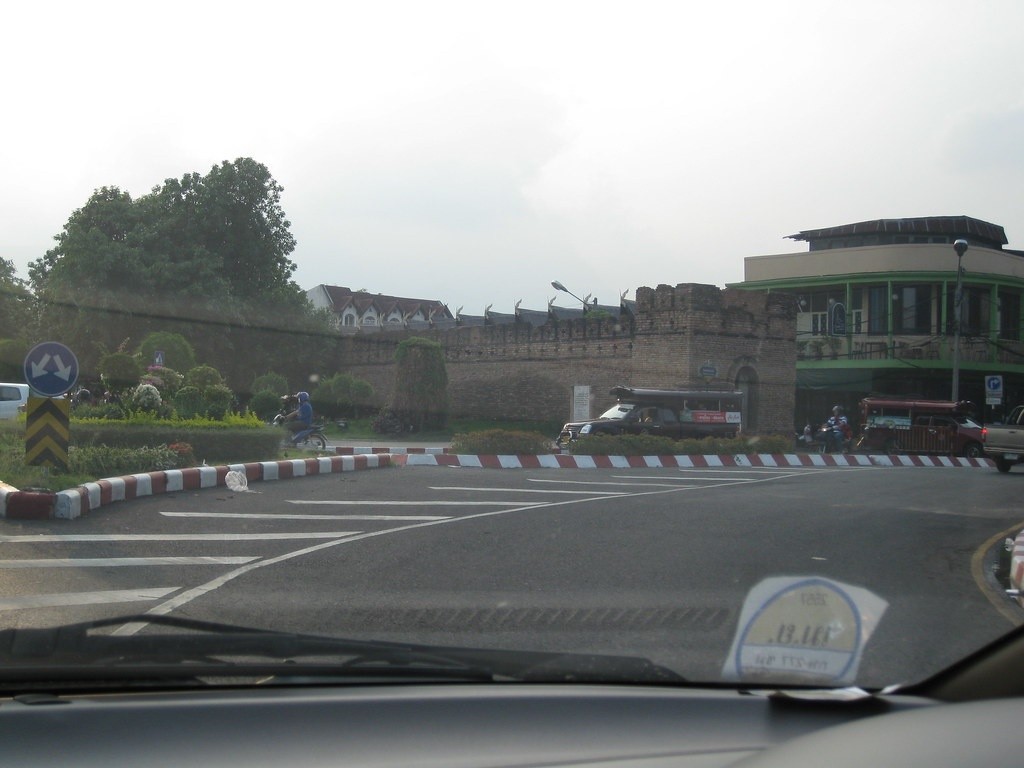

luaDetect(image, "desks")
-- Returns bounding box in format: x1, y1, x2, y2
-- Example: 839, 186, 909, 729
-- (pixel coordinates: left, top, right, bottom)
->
865, 342, 888, 359
911, 347, 924, 359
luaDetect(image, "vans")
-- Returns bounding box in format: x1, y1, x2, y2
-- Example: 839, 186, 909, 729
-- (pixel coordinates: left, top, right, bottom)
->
556, 387, 744, 446
860, 397, 983, 459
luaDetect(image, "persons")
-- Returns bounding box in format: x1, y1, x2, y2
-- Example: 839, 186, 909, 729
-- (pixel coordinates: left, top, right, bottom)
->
822, 406, 848, 452
280, 392, 312, 432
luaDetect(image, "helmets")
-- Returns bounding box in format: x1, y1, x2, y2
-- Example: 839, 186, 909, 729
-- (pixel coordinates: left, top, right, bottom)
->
832, 405, 843, 415
295, 391, 310, 402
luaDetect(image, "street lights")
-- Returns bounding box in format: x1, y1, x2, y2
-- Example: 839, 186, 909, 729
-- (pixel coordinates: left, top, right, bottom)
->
953, 239, 969, 402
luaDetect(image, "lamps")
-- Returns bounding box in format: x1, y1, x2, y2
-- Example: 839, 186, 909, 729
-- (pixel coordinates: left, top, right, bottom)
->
800, 296, 807, 306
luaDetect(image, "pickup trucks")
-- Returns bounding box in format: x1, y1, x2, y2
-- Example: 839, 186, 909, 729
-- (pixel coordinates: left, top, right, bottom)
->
982, 406, 1024, 473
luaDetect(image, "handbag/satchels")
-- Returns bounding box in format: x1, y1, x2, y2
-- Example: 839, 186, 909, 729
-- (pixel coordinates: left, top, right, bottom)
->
836, 416, 853, 439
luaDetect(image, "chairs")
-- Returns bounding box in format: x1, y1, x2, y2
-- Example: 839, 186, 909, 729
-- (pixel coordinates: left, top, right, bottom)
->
879, 340, 895, 359
898, 341, 910, 356
926, 339, 941, 359
852, 342, 864, 359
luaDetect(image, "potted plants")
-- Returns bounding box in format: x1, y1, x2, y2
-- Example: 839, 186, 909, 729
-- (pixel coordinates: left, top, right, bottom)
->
823, 337, 842, 360
796, 341, 810, 360
809, 341, 826, 362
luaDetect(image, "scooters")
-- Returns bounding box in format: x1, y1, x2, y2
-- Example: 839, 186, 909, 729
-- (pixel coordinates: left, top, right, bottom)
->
274, 414, 327, 450
813, 422, 857, 456
